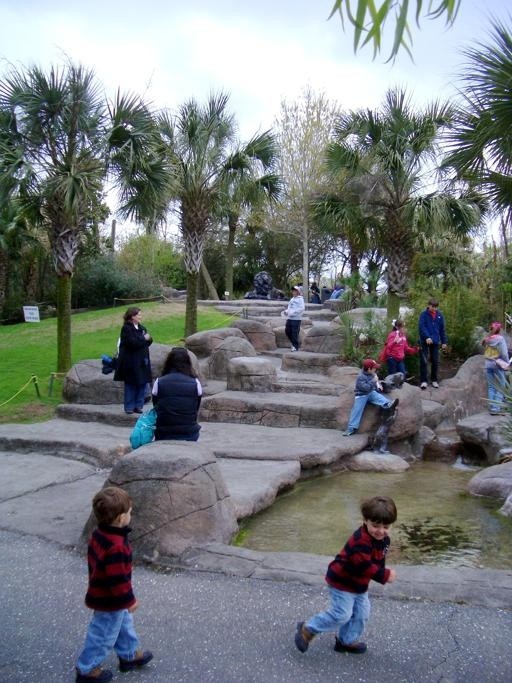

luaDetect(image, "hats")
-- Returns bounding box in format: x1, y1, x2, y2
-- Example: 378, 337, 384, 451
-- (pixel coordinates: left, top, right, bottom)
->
294, 285, 303, 293
362, 359, 381, 370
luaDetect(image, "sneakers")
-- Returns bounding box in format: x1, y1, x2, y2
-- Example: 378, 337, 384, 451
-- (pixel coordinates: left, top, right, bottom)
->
119, 651, 153, 673
296, 621, 312, 653
74, 666, 112, 683
420, 381, 428, 390
386, 397, 399, 411
341, 428, 356, 436
291, 346, 297, 351
135, 407, 142, 412
431, 382, 439, 388
333, 636, 367, 653
124, 408, 133, 414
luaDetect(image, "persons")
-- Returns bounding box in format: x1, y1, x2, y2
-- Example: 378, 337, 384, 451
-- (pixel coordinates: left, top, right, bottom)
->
323, 285, 327, 288
283, 284, 306, 353
310, 279, 322, 303
481, 322, 512, 416
298, 282, 304, 298
340, 357, 400, 437
78, 487, 158, 656
330, 273, 346, 300
387, 318, 421, 377
110, 306, 160, 415
418, 298, 443, 390
151, 346, 203, 442
294, 494, 399, 653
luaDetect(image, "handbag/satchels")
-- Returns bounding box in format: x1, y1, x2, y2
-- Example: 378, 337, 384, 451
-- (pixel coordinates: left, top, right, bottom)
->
495, 356, 512, 372
130, 407, 158, 449
